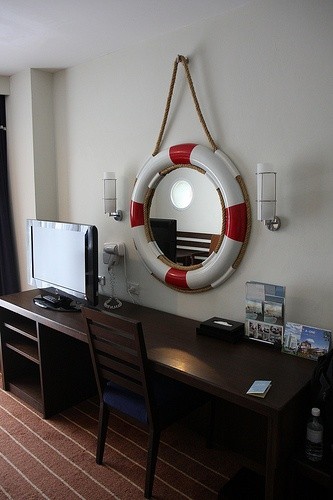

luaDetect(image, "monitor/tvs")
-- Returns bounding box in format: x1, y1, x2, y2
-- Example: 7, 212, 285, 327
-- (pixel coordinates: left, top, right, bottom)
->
25, 219, 98, 312
150, 218, 178, 263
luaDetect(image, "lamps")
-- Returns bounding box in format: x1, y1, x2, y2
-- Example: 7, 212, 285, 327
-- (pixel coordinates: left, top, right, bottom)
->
103, 170, 124, 220
257, 163, 281, 232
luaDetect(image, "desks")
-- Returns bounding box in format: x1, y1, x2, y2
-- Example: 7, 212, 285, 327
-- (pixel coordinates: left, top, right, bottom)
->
0, 288, 318, 500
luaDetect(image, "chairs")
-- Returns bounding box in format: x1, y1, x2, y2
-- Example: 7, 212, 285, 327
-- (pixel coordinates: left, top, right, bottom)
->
78, 304, 198, 499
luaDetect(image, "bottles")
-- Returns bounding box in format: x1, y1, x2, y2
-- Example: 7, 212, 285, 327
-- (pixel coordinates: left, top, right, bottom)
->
304, 408, 324, 461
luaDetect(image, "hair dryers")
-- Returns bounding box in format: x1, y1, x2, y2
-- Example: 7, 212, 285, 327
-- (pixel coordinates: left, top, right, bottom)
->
105, 245, 121, 272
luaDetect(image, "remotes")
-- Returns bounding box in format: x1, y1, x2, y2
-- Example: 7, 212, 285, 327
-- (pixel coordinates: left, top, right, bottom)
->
43, 296, 59, 304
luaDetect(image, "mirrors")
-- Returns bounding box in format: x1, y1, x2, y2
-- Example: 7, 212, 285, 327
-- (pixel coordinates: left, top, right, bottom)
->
128, 54, 251, 295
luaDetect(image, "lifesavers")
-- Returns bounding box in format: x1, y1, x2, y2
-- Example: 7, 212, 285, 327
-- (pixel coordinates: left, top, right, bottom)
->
129, 143, 246, 290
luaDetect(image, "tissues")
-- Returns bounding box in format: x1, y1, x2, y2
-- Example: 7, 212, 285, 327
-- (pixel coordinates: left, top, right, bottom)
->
199, 316, 246, 340
